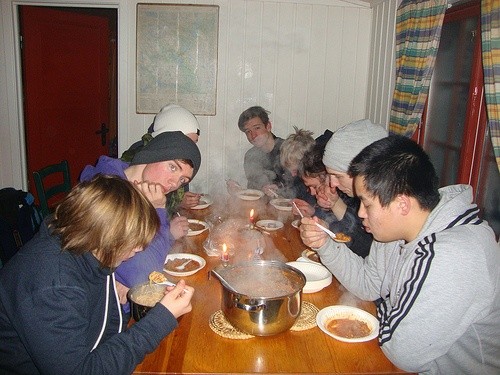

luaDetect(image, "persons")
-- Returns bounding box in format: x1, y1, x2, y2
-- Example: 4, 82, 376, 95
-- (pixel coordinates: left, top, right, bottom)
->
300, 134, 500, 375
227, 106, 285, 195
315, 119, 388, 258
80, 131, 200, 325
261, 125, 317, 203
0, 172, 195, 374
287, 129, 334, 220
121, 105, 201, 218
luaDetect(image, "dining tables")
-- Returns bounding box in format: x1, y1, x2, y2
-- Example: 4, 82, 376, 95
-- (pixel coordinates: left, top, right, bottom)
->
125, 193, 417, 375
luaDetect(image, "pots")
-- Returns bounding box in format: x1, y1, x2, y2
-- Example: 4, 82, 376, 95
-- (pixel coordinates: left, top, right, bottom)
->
217, 260, 307, 337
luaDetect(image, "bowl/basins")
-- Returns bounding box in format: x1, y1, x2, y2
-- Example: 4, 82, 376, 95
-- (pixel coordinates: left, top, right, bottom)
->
128, 280, 158, 322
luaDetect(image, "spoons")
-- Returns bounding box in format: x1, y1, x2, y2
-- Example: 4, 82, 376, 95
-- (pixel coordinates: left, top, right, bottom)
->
269, 189, 279, 198
176, 260, 191, 270
154, 277, 189, 293
315, 223, 351, 242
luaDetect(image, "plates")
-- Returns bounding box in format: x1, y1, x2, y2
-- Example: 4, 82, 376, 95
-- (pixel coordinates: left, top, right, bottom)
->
190, 198, 215, 209
237, 189, 264, 200
162, 254, 207, 276
270, 199, 293, 210
285, 261, 333, 293
256, 219, 285, 231
316, 305, 380, 342
185, 219, 209, 236
291, 219, 300, 230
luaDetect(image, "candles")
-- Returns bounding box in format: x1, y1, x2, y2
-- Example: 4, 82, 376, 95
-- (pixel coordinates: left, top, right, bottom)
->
222, 243, 228, 260
249, 208, 255, 224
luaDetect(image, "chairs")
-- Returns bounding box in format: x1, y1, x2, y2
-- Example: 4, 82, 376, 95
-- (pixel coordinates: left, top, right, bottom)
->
0, 187, 40, 267
33, 160, 72, 221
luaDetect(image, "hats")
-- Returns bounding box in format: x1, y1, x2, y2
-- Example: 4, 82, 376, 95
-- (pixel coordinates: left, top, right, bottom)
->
151, 104, 200, 137
322, 118, 388, 173
135, 131, 202, 186
